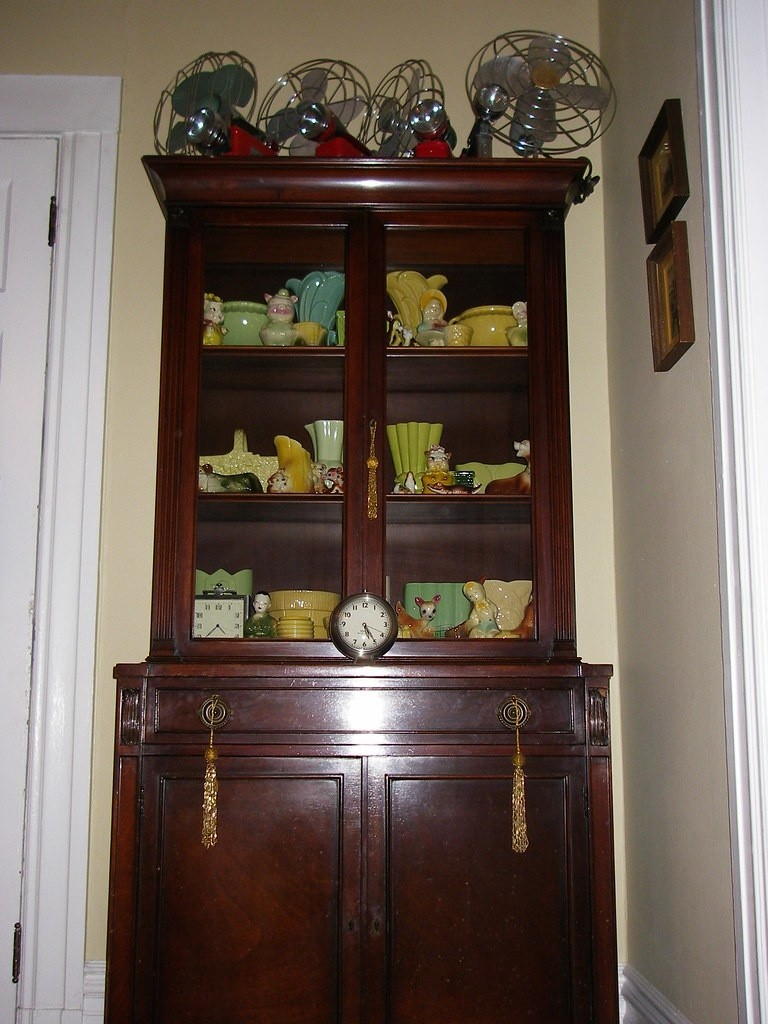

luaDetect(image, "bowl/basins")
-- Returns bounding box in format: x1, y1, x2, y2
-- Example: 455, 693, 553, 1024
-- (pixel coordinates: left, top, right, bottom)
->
221, 300, 269, 346
449, 305, 517, 346
268, 590, 340, 639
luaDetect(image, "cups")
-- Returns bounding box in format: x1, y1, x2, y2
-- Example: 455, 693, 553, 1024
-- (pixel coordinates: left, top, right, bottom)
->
445, 324, 475, 345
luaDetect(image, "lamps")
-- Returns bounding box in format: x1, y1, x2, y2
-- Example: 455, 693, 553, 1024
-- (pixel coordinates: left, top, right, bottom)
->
408, 99, 457, 158
299, 104, 372, 157
186, 105, 278, 158
459, 84, 510, 158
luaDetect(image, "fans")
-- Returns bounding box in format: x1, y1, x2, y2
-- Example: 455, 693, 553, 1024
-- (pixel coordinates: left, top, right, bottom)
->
255, 58, 372, 155
464, 29, 618, 160
152, 50, 258, 163
361, 59, 445, 158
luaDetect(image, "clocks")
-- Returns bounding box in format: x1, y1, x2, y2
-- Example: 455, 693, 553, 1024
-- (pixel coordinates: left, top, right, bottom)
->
195, 594, 248, 639
329, 592, 398, 659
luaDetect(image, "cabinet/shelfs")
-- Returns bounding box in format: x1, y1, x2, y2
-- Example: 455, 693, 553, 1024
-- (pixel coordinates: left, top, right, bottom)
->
140, 153, 579, 664
103, 661, 620, 1024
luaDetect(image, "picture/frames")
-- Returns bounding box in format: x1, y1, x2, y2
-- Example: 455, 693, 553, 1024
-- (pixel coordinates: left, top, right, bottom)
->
645, 221, 695, 372
638, 99, 690, 244
